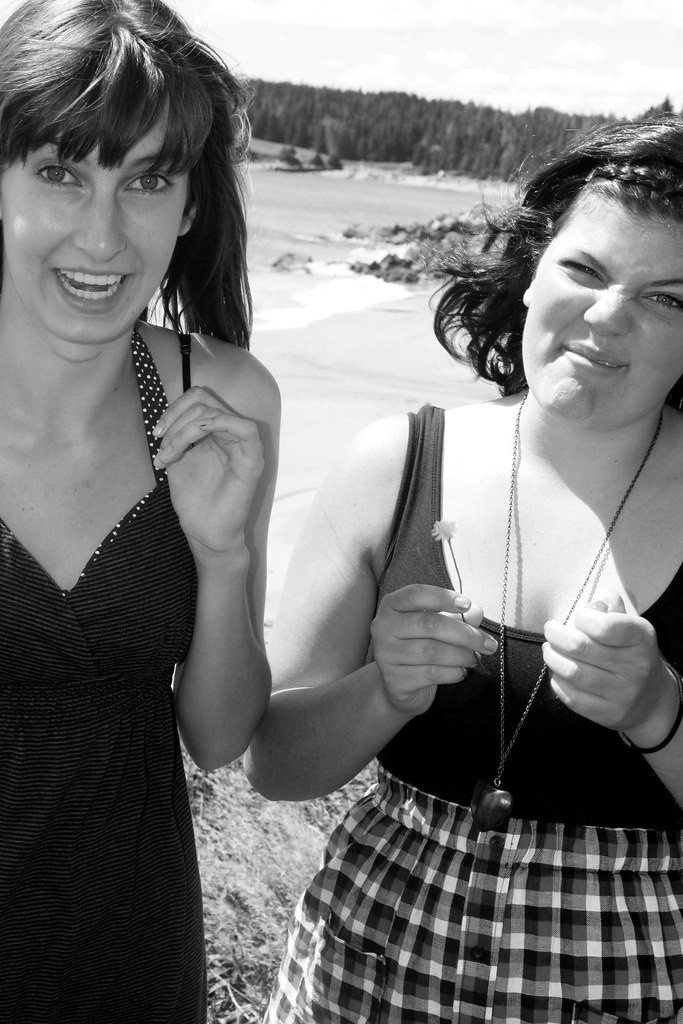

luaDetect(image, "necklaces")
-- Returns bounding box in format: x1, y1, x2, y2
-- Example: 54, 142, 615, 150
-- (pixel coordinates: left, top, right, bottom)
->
474, 386, 672, 834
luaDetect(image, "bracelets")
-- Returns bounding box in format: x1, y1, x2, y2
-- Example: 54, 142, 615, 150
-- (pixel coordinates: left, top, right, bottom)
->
620, 658, 683, 754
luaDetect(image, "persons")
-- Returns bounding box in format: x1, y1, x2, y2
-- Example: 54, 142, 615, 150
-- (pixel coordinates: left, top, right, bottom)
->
243, 114, 683, 1024
0, 0, 281, 1024
